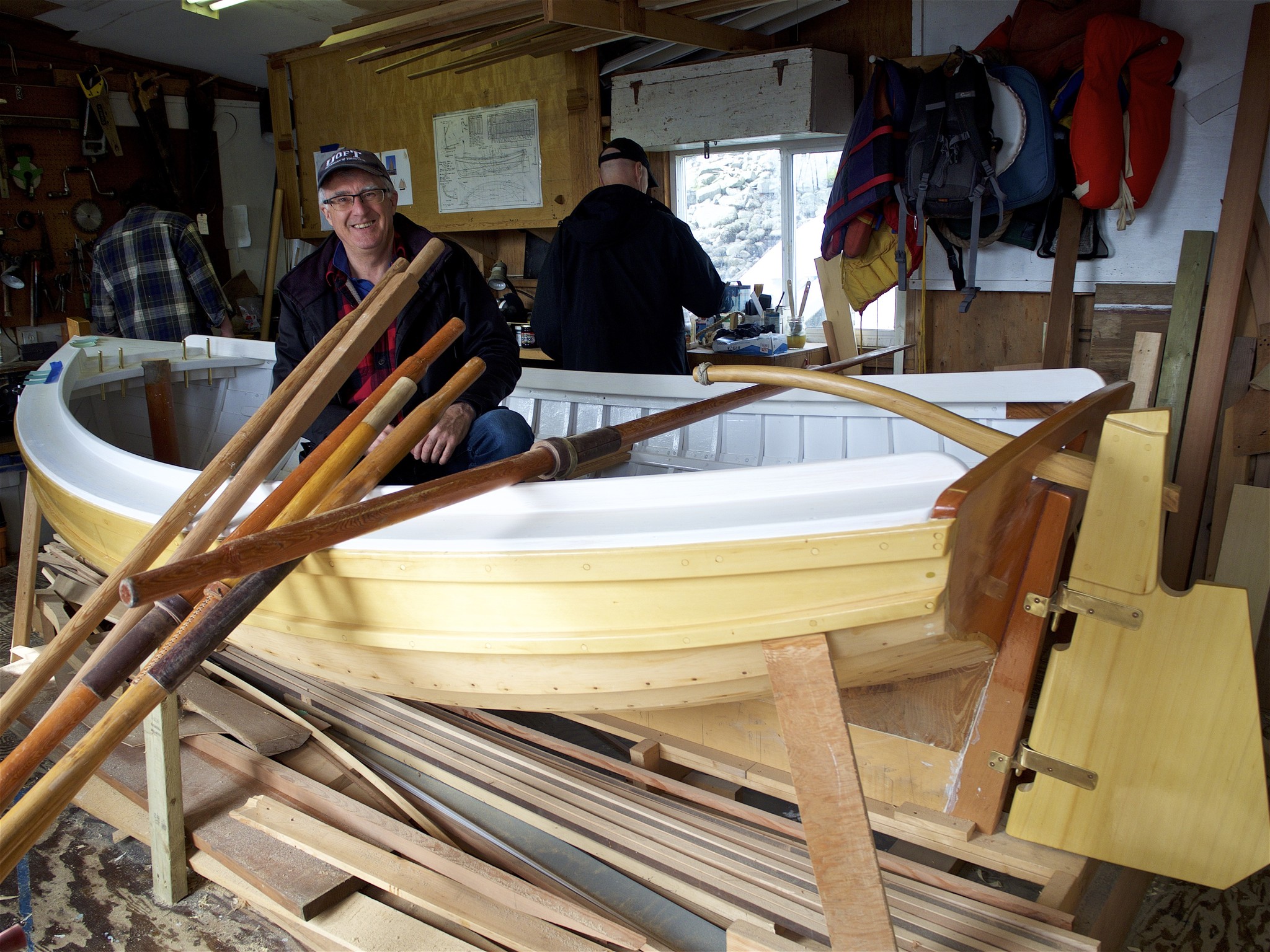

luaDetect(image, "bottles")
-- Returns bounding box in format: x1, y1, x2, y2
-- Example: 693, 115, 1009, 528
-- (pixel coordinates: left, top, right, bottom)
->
785, 315, 806, 349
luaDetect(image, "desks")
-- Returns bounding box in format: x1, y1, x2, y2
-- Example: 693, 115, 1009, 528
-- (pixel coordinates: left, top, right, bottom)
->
515, 343, 829, 367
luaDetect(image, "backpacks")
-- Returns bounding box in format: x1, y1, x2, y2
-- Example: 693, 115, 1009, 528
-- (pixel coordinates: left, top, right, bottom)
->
894, 49, 1007, 313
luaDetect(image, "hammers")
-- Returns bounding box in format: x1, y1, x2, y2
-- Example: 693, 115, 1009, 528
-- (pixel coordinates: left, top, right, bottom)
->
0, 251, 13, 317
25, 249, 49, 326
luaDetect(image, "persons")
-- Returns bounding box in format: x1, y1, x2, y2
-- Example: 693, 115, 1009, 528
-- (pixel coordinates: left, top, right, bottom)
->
725, 291, 736, 311
533, 137, 725, 376
270, 149, 534, 484
92, 170, 235, 342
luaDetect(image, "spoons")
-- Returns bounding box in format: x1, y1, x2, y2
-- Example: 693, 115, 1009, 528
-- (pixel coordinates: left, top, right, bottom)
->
787, 279, 811, 335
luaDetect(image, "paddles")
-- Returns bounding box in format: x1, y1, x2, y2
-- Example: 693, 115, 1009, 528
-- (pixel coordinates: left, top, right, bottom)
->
1, 237, 486, 885
118, 345, 917, 609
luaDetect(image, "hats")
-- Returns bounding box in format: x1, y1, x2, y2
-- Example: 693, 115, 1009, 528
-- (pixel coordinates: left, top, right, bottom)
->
598, 138, 659, 189
318, 149, 393, 191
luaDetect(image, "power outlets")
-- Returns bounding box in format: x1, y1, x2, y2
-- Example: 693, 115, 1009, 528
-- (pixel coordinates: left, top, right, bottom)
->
22, 331, 38, 345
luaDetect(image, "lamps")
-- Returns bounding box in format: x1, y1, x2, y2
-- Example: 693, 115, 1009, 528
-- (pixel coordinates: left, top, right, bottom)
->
180, 0, 258, 21
488, 260, 527, 322
0, 256, 26, 290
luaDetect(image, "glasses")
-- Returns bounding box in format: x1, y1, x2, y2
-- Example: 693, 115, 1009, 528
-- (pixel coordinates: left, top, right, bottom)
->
323, 189, 389, 211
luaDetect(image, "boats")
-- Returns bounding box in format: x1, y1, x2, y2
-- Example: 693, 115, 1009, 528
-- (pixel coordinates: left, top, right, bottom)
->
13, 333, 1111, 717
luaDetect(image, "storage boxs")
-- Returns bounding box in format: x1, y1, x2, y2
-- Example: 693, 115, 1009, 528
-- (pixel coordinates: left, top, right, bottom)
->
711, 332, 788, 356
611, 48, 855, 148
